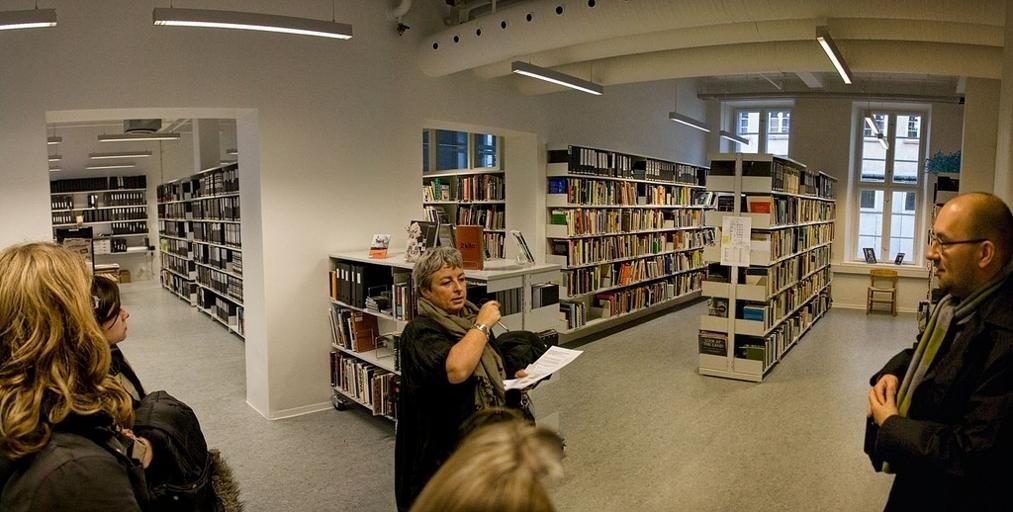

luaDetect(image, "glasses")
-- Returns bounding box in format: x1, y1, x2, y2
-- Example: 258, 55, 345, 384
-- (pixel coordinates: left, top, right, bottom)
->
927, 229, 984, 251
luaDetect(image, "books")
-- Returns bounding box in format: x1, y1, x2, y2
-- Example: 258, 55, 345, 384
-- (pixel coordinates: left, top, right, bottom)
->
546, 146, 717, 328
423, 174, 504, 259
326, 261, 417, 419
51, 169, 243, 337
697, 160, 836, 370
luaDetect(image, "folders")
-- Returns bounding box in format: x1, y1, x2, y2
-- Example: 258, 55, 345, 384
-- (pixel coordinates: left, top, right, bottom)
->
335, 261, 388, 309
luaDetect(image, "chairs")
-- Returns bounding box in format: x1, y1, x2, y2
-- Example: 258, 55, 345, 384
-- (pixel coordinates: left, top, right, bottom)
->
867, 269, 898, 316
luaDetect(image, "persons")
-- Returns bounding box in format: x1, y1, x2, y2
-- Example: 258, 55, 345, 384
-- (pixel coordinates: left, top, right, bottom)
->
0, 242, 150, 512
867, 191, 1013, 512
90, 275, 153, 470
407, 406, 567, 512
394, 247, 528, 512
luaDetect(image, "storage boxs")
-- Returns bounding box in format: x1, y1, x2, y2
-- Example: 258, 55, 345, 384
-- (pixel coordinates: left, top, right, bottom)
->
119, 270, 131, 283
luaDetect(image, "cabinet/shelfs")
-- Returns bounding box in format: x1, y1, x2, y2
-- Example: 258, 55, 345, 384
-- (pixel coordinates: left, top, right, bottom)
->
50, 189, 151, 293
423, 166, 504, 258
157, 161, 243, 340
699, 154, 837, 383
918, 173, 961, 332
329, 254, 561, 435
547, 145, 710, 345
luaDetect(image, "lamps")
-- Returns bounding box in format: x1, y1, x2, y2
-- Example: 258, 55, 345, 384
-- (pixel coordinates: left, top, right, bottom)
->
47, 119, 238, 171
512, 56, 604, 95
152, 0, 352, 40
816, 26, 889, 149
720, 94, 749, 145
0, 0, 58, 30
669, 83, 712, 132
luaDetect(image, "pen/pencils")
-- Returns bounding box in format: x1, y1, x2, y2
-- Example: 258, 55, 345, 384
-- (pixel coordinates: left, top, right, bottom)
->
498, 321, 510, 332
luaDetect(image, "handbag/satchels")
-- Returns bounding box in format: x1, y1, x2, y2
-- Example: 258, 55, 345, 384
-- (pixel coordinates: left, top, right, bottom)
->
520, 392, 536, 424
